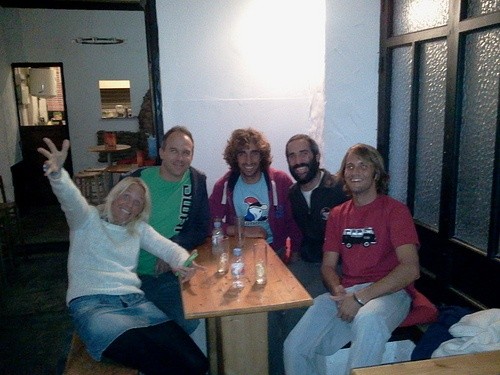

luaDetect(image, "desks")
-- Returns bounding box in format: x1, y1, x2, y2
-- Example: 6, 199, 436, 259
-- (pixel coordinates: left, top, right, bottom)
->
85, 144, 131, 198
350, 349, 500, 375
177, 227, 315, 375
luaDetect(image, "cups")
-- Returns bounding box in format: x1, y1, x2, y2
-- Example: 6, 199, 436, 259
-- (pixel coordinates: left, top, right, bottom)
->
253, 243, 268, 285
103, 134, 116, 150
232, 216, 246, 247
212, 236, 230, 273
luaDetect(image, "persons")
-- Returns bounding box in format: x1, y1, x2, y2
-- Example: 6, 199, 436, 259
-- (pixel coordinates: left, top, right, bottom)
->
37, 137, 207, 375
208, 129, 302, 265
267, 134, 350, 375
116, 125, 208, 336
283, 143, 419, 375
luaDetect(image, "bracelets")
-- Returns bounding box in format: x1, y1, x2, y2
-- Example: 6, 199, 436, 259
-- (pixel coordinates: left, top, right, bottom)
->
353, 293, 365, 306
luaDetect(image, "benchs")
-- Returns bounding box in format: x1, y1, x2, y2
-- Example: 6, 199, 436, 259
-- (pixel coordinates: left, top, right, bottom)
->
107, 162, 137, 182
62, 325, 140, 375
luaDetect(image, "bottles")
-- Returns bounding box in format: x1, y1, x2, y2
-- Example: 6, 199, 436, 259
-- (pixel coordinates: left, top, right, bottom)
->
230, 247, 245, 288
212, 222, 224, 235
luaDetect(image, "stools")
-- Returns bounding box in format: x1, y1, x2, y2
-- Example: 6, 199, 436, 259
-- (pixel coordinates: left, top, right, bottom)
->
83, 165, 107, 205
0, 200, 28, 285
73, 170, 105, 208
